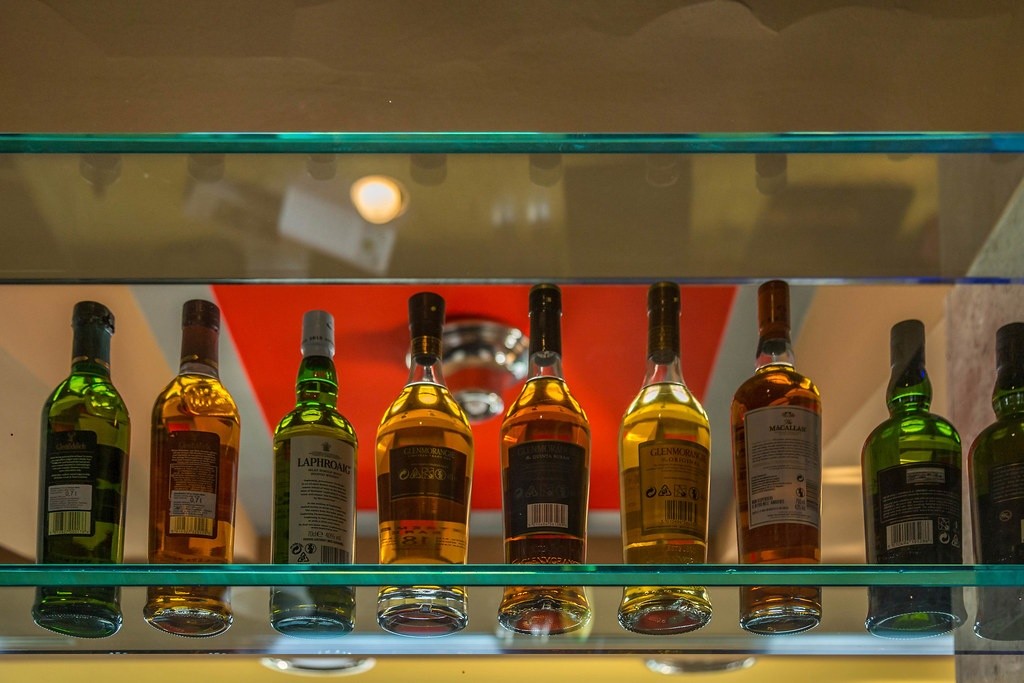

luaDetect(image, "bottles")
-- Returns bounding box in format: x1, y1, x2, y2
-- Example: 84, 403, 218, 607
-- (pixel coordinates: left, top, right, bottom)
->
968, 319, 1024, 641
31, 300, 132, 639
618, 281, 714, 636
496, 283, 592, 636
861, 319, 968, 642
268, 310, 359, 640
373, 292, 475, 640
729, 280, 824, 638
143, 299, 241, 640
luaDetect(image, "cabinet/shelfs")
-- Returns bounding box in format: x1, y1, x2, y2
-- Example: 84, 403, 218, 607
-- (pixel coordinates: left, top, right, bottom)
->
0, 131, 1024, 660
